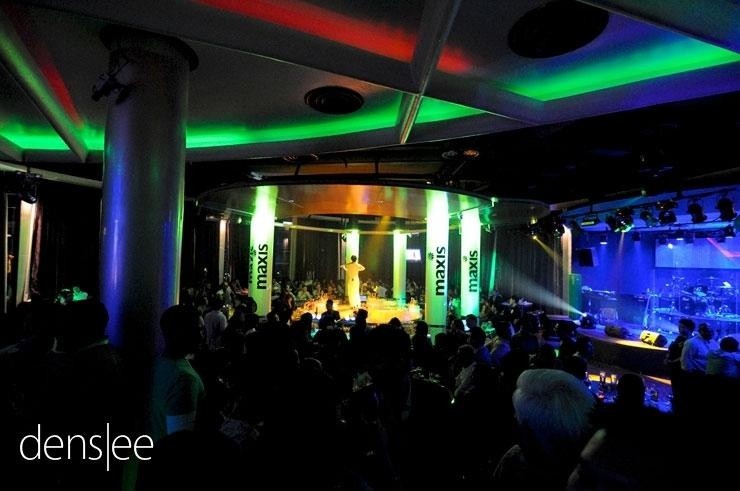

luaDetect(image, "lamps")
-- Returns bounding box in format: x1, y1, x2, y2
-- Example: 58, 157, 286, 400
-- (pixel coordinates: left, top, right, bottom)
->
578, 311, 600, 332
523, 187, 739, 237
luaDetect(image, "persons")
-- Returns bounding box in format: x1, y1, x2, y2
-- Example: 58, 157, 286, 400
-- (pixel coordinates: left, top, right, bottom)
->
339, 253, 366, 312
1, 275, 740, 491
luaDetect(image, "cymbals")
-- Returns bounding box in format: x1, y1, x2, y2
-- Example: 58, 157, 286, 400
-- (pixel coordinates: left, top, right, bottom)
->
706, 277, 720, 280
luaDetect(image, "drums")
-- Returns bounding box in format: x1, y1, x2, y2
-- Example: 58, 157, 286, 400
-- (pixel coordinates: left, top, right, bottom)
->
682, 296, 707, 313
